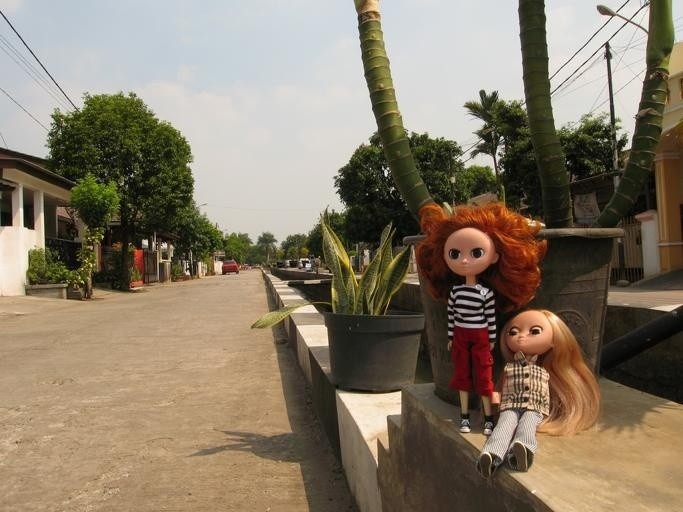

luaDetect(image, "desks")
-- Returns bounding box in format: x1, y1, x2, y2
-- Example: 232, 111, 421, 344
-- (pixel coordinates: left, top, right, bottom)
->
596, 5, 648, 38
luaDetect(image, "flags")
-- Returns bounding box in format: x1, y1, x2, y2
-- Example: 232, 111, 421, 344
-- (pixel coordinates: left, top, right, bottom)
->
401, 227, 625, 412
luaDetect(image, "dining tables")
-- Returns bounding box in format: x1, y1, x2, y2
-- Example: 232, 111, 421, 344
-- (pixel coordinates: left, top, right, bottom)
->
250, 201, 425, 395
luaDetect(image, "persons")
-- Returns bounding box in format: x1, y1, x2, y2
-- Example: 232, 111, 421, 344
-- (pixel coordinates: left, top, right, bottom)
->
359, 252, 366, 272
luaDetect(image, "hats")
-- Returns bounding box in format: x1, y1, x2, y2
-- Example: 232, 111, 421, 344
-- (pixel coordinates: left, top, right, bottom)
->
222, 259, 239, 274
